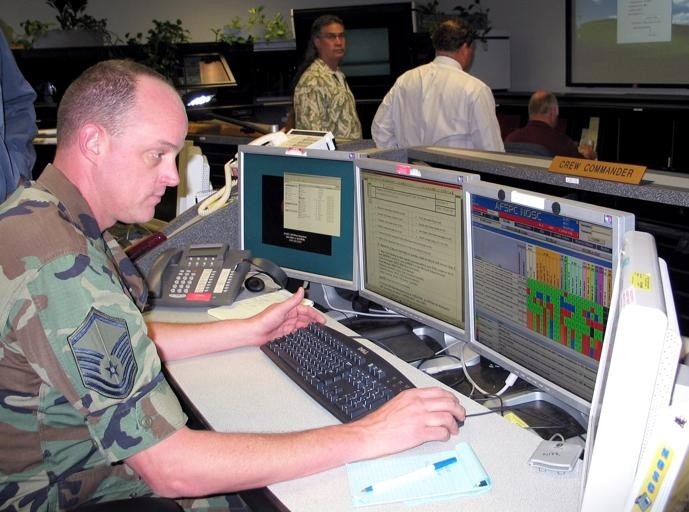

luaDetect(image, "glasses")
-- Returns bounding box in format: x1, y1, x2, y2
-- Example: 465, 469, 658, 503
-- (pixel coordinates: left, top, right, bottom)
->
322, 32, 345, 40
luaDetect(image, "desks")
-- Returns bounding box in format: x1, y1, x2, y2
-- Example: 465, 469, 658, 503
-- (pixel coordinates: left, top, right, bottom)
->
133, 277, 584, 512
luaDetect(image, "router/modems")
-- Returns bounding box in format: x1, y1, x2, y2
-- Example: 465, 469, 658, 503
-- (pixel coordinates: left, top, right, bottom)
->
530, 440, 583, 474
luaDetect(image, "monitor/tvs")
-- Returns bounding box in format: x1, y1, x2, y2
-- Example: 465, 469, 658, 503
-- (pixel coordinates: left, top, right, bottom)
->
462, 181, 635, 451
353, 157, 481, 375
161, 52, 238, 91
175, 140, 213, 218
566, 0, 689, 88
578, 229, 689, 512
237, 145, 368, 321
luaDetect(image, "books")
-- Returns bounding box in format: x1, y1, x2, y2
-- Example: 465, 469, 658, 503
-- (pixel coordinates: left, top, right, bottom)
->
206, 288, 315, 320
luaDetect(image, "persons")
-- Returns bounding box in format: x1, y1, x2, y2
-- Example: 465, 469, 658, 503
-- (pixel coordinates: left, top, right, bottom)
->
502, 87, 596, 161
1, 59, 468, 510
1, 33, 38, 202
291, 16, 364, 145
370, 15, 510, 157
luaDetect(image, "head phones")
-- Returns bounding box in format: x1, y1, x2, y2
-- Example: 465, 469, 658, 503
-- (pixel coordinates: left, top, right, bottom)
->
234, 255, 288, 295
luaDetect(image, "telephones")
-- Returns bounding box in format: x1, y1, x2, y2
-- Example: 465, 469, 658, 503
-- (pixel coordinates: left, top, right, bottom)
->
147, 243, 251, 306
230, 128, 338, 177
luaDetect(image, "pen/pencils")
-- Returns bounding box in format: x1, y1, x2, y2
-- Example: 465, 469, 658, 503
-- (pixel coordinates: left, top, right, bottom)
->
361, 457, 457, 493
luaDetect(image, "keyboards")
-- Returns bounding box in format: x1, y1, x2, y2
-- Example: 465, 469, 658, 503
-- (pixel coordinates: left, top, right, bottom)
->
260, 322, 417, 426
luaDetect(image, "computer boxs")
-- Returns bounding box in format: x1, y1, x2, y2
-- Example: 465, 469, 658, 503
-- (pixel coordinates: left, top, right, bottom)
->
152, 131, 252, 224
